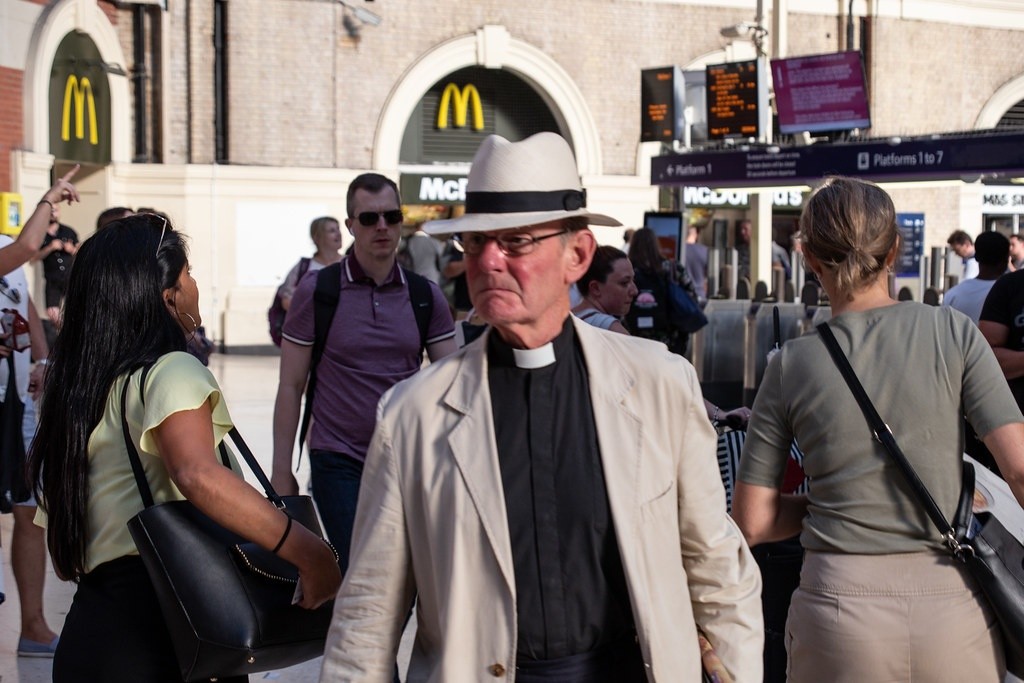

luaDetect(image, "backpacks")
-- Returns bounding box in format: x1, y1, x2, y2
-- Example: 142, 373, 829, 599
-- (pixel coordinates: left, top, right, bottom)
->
269, 258, 313, 346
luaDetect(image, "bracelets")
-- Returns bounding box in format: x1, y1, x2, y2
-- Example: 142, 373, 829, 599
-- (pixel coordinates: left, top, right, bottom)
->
37, 200, 52, 210
270, 510, 293, 558
714, 407, 719, 421
35, 359, 51, 367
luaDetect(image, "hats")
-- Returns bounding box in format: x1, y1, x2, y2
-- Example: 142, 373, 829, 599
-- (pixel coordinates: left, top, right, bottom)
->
421, 131, 624, 241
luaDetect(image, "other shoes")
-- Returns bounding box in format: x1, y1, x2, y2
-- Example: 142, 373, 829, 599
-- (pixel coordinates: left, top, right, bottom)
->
17, 635, 60, 657
0, 592, 6, 604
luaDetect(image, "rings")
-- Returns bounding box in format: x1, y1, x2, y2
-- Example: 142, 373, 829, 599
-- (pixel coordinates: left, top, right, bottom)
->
746, 415, 751, 417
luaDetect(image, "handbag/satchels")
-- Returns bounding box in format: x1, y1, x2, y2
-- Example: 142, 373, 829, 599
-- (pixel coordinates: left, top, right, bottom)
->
712, 418, 811, 518
666, 263, 709, 334
122, 356, 347, 683
954, 510, 1024, 680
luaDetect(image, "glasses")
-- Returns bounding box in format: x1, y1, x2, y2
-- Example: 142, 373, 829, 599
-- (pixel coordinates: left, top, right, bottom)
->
461, 230, 568, 256
353, 210, 403, 226
142, 212, 170, 256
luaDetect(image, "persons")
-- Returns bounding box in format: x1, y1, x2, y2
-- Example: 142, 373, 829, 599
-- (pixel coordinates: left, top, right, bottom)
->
270, 173, 459, 560
34, 209, 344, 683
0, 166, 1024, 660
731, 177, 1024, 683
322, 130, 765, 683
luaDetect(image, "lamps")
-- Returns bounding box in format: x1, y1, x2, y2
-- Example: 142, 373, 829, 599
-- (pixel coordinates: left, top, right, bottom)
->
339, 1, 382, 27
